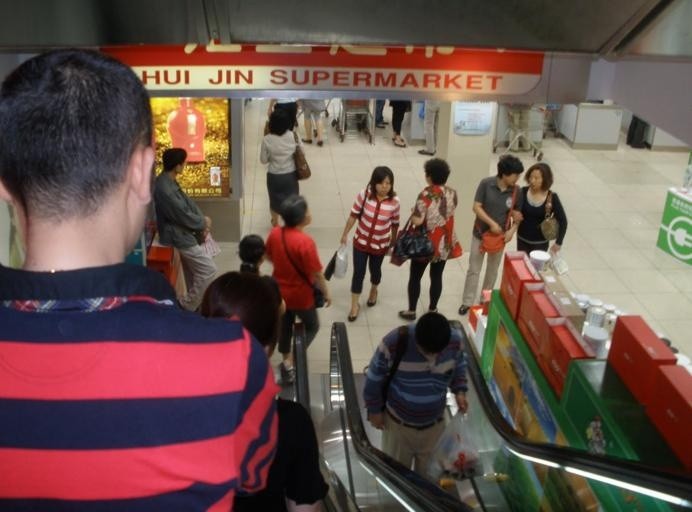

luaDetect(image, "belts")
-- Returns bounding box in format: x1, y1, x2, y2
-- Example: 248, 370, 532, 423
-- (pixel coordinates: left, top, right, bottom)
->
382, 404, 447, 432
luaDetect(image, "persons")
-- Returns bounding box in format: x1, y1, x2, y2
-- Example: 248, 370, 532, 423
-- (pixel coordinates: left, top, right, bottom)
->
260, 111, 305, 229
2, 46, 279, 512
362, 313, 469, 476
340, 154, 567, 321
201, 195, 333, 511
268, 98, 440, 157
157, 146, 218, 302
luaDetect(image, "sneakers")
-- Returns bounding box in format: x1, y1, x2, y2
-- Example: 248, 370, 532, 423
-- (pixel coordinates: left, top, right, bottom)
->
396, 309, 418, 321
416, 146, 438, 157
457, 304, 471, 315
391, 136, 409, 148
366, 289, 379, 307
302, 137, 313, 144
317, 140, 324, 147
346, 302, 361, 322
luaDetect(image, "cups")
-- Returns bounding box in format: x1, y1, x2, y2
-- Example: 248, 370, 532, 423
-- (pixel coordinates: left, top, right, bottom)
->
572, 293, 628, 352
529, 250, 550, 272
210, 166, 221, 187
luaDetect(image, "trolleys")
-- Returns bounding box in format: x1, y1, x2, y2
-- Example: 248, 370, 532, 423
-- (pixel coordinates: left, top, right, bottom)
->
493, 104, 550, 160
295, 98, 376, 146
543, 102, 565, 138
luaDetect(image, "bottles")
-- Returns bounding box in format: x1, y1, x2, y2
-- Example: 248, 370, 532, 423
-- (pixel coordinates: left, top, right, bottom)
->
166, 97, 207, 164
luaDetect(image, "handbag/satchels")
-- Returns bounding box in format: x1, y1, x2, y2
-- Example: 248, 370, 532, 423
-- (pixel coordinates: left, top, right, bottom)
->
480, 229, 506, 254
539, 192, 561, 242
312, 288, 325, 309
362, 321, 410, 423
292, 131, 312, 181
390, 212, 435, 268
445, 237, 463, 261
320, 243, 350, 283
